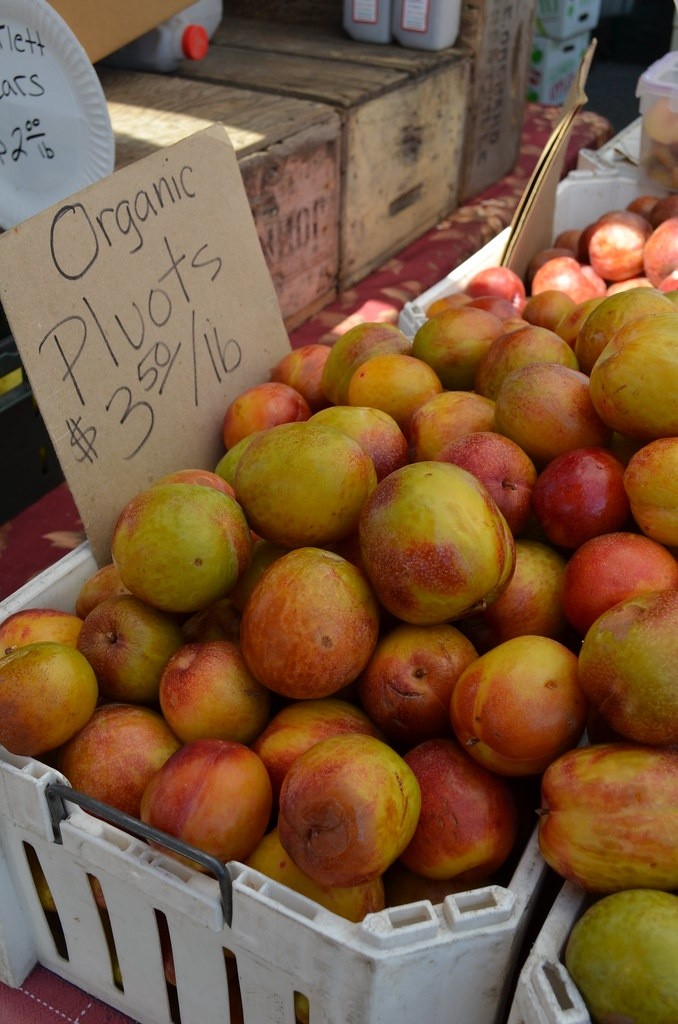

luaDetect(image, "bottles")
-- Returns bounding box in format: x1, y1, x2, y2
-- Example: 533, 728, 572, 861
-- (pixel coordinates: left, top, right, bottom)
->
341, 0, 395, 44
102, 0, 226, 71
391, 0, 465, 52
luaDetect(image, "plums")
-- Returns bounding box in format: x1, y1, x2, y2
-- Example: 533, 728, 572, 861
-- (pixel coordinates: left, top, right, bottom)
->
0, 194, 678, 1024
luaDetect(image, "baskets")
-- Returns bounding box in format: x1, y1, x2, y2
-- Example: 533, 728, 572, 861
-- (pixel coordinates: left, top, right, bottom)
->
0, 534, 556, 1024
508, 867, 590, 1023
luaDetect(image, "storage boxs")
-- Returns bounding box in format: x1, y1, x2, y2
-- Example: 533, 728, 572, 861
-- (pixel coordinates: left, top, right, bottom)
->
1, 0, 602, 1024
